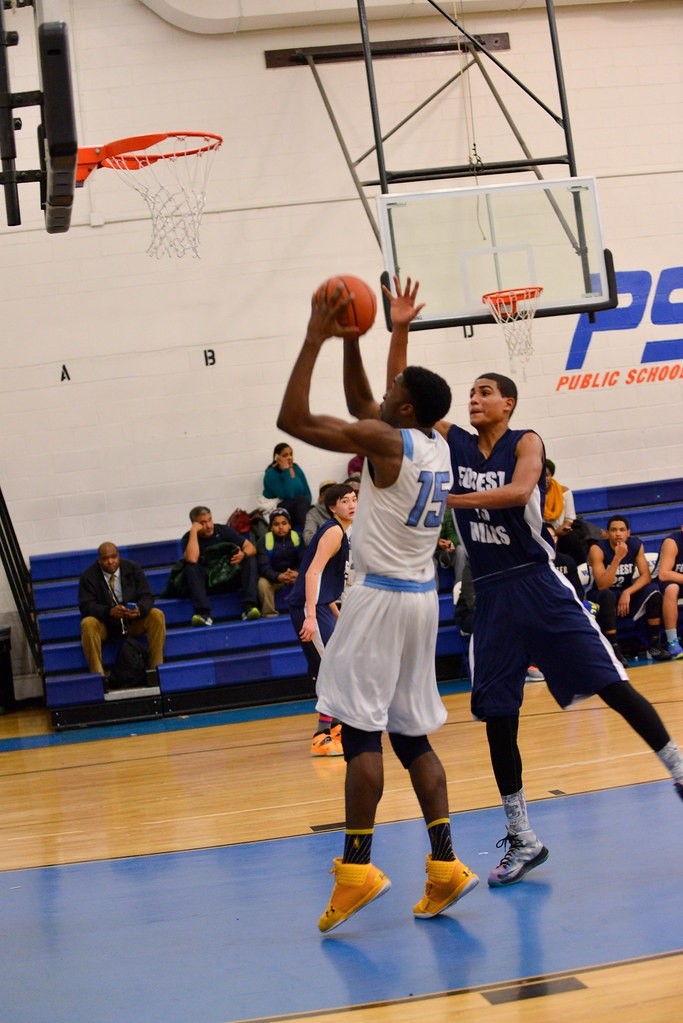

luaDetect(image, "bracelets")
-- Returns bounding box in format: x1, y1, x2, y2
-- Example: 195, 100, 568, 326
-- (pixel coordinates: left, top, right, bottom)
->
243, 551, 247, 560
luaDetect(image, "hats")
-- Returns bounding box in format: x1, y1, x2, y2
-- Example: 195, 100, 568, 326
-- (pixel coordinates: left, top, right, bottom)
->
269, 509, 291, 528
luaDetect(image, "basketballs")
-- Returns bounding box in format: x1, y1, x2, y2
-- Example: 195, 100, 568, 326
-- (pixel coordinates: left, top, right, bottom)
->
313, 275, 377, 337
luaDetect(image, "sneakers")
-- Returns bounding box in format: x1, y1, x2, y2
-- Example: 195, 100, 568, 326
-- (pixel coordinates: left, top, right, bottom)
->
612, 643, 628, 666
645, 648, 671, 660
486, 828, 550, 885
411, 851, 479, 920
666, 639, 683, 659
317, 853, 390, 933
311, 725, 344, 756
191, 615, 213, 627
524, 666, 546, 681
242, 607, 260, 621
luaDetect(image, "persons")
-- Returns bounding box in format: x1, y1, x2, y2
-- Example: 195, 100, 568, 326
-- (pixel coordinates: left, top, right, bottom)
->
545, 458, 576, 542
284, 483, 343, 758
584, 515, 671, 667
276, 281, 479, 933
381, 273, 683, 888
303, 479, 337, 546
256, 508, 307, 619
262, 442, 312, 532
543, 522, 585, 605
181, 505, 261, 627
649, 523, 683, 660
348, 454, 365, 478
437, 505, 467, 605
449, 580, 545, 688
79, 541, 166, 694
342, 477, 361, 499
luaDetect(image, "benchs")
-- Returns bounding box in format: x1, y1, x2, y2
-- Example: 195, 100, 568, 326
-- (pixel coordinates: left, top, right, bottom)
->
27, 476, 683, 733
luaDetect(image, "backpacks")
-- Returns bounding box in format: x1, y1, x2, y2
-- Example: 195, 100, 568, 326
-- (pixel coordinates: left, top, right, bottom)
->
110, 638, 150, 687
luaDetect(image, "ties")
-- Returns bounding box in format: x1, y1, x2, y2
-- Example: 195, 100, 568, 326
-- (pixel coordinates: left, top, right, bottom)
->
111, 574, 116, 606
